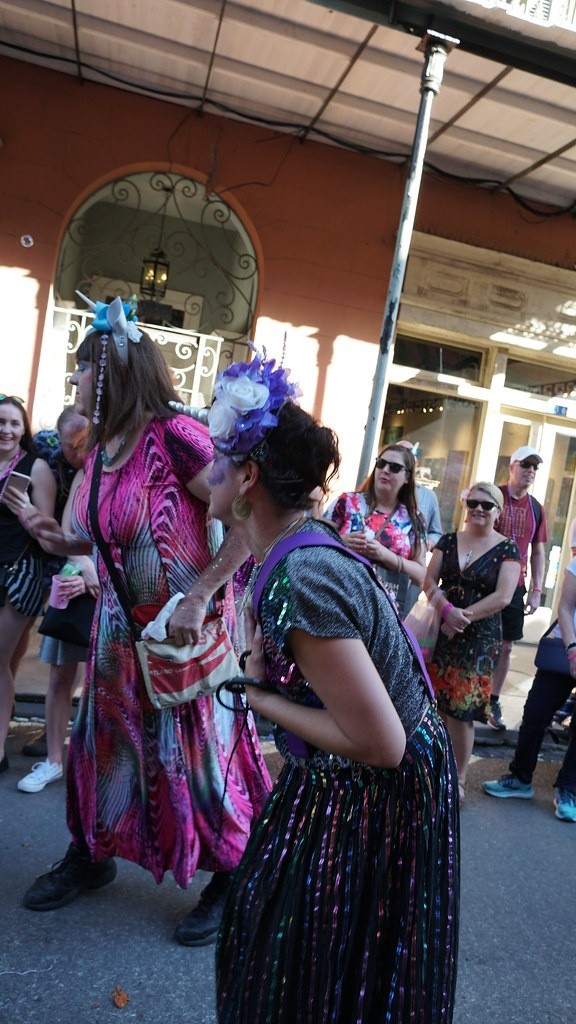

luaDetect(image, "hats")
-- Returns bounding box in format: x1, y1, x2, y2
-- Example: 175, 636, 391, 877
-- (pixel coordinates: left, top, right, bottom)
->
510, 446, 543, 465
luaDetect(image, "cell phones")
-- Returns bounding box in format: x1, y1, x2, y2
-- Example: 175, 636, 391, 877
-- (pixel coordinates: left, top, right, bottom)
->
0, 472, 32, 503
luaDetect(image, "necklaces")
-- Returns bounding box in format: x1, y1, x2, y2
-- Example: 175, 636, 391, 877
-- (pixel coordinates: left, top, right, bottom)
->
0, 449, 22, 499
238, 517, 302, 616
100, 428, 133, 467
507, 482, 536, 559
465, 550, 473, 564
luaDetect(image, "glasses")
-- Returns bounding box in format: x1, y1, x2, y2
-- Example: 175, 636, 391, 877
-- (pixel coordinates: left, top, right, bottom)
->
0, 394, 24, 403
376, 457, 409, 473
514, 461, 538, 470
466, 499, 500, 510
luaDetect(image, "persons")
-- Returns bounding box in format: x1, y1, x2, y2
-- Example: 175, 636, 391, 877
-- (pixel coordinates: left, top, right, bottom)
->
205, 339, 463, 1024
424, 482, 522, 810
396, 440, 443, 550
486, 445, 548, 730
481, 515, 576, 823
311, 444, 427, 619
0, 393, 100, 792
22, 297, 274, 945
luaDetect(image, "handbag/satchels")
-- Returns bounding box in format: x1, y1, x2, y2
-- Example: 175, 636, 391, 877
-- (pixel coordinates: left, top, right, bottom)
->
403, 586, 445, 662
534, 635, 571, 672
136, 618, 246, 709
37, 572, 97, 648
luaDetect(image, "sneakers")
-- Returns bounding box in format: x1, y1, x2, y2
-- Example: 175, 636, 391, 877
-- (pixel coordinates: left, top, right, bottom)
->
483, 772, 533, 798
486, 709, 506, 731
553, 788, 576, 822
175, 872, 234, 945
18, 757, 64, 793
23, 842, 117, 910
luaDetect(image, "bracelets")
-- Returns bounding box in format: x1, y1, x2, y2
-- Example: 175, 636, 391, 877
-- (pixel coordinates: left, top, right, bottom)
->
533, 588, 542, 592
568, 652, 576, 660
441, 603, 453, 620
566, 642, 576, 652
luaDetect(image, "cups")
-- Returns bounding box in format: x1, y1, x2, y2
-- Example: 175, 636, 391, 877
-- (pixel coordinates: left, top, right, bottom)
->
48, 574, 73, 609
